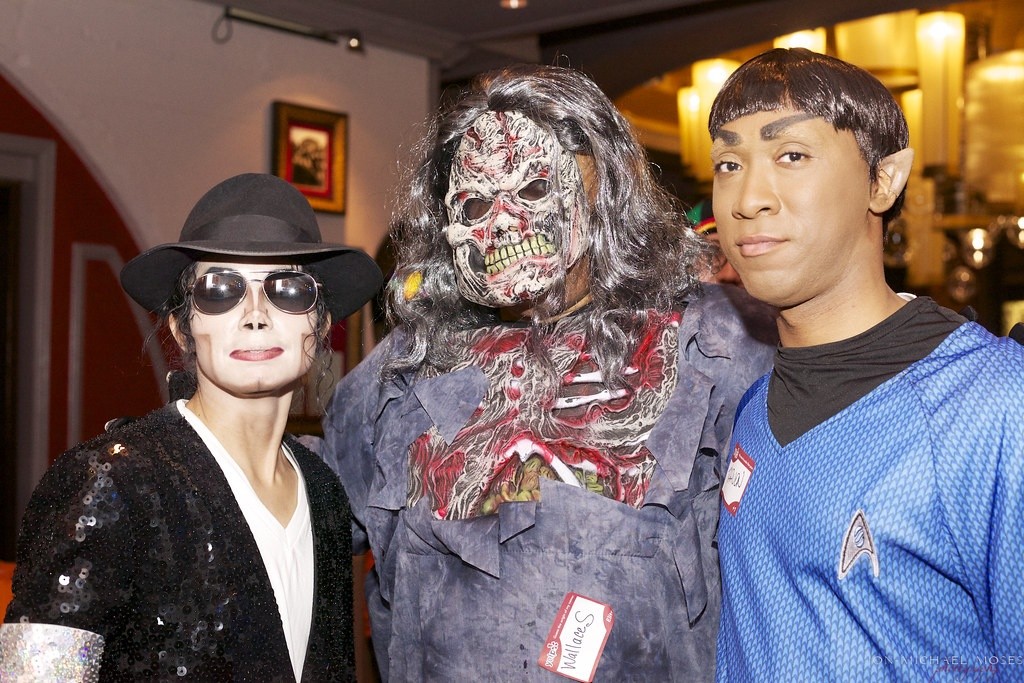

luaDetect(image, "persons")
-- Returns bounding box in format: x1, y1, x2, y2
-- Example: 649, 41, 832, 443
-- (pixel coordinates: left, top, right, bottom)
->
0, 173, 381, 683
715, 47, 1024, 683
303, 63, 779, 683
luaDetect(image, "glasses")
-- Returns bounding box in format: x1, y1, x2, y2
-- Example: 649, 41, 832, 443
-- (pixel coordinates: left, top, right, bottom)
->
186, 272, 323, 314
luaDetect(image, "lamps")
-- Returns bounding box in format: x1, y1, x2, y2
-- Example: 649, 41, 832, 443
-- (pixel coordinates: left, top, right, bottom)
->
678, 0, 964, 180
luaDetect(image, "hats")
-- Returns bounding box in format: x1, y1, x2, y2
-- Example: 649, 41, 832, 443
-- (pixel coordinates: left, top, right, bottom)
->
120, 173, 384, 324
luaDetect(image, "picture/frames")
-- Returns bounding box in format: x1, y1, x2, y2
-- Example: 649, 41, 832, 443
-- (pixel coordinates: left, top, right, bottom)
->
270, 100, 350, 215
